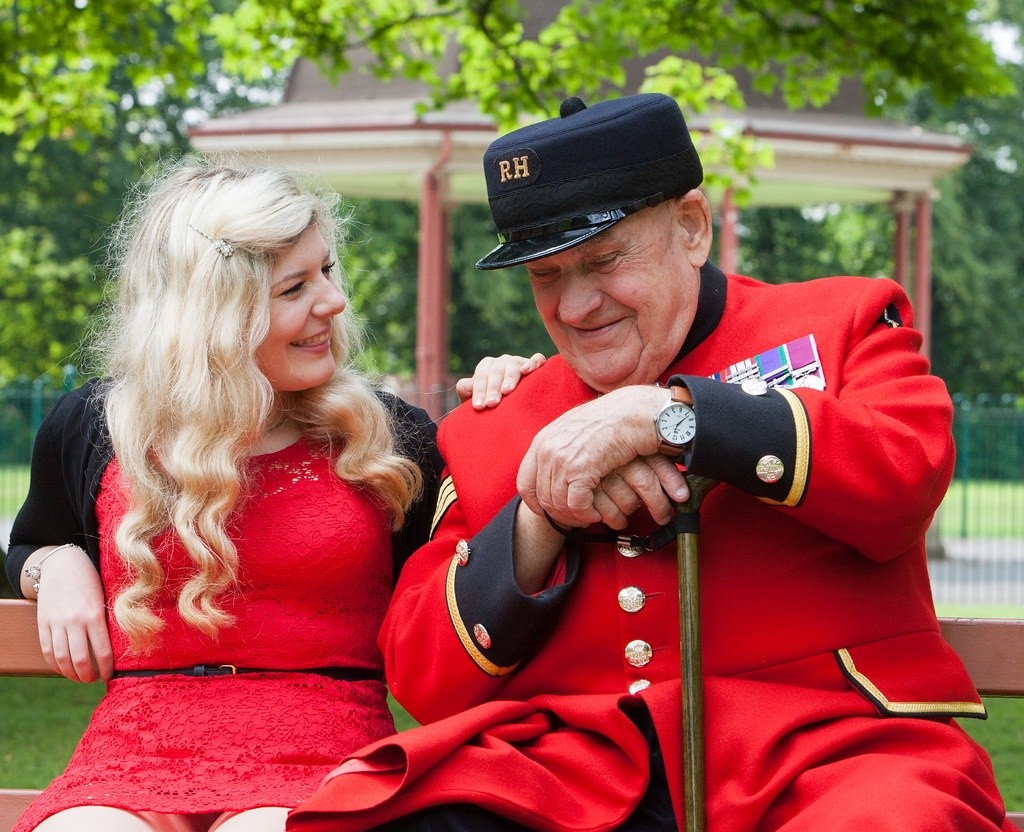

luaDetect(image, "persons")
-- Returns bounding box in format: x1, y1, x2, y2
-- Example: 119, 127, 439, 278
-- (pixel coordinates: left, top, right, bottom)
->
284, 92, 1013, 832
3, 161, 547, 832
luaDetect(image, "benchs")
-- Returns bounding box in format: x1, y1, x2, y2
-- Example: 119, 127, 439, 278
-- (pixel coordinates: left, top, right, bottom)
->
0, 595, 1022, 832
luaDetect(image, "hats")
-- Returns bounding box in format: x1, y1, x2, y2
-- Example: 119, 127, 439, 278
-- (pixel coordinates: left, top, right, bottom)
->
474, 91, 703, 271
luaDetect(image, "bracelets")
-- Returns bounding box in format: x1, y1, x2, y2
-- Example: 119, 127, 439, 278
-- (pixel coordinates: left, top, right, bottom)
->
23, 543, 82, 597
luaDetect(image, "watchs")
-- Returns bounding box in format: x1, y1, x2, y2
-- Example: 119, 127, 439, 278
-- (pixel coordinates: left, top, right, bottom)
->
654, 386, 696, 456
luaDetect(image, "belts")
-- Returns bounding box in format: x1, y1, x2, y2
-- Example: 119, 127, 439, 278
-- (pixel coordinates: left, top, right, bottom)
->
112, 661, 382, 679
